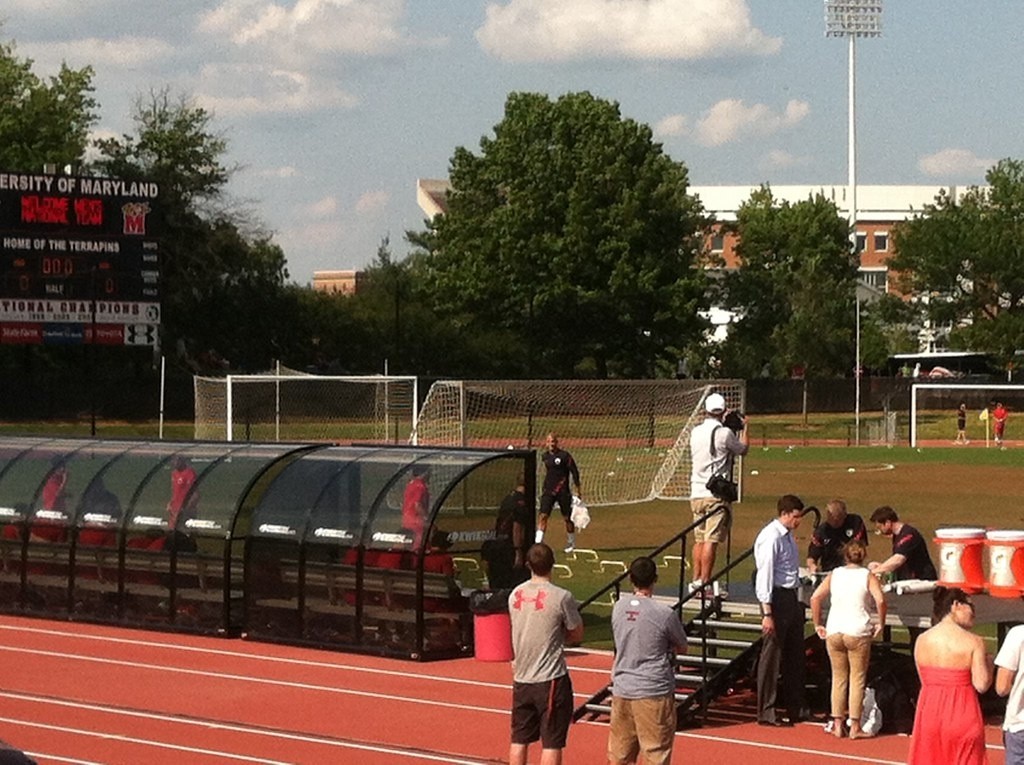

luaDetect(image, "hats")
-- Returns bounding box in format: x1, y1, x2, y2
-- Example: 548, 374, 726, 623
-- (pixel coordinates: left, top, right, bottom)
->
705, 393, 725, 415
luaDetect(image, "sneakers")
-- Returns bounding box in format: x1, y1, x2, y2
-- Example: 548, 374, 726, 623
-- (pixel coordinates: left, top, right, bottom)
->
563, 541, 575, 554
688, 578, 729, 600
391, 631, 415, 642
374, 630, 392, 642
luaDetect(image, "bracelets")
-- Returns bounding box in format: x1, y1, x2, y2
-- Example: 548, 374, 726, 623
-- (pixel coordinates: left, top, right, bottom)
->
514, 546, 522, 550
763, 613, 772, 616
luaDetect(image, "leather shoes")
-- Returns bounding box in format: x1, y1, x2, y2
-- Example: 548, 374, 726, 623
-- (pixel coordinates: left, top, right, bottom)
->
790, 714, 823, 722
759, 719, 793, 727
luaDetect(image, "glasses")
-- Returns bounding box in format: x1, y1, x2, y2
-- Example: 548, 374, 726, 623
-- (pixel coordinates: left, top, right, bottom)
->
963, 602, 975, 613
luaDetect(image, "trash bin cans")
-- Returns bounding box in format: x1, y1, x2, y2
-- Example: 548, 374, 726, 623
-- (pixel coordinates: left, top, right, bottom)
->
471, 590, 514, 661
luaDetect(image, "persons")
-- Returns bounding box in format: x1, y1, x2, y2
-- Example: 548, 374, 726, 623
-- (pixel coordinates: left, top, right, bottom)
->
909, 586, 995, 765
754, 495, 827, 726
79, 477, 123, 519
690, 392, 749, 599
869, 506, 937, 644
810, 540, 887, 738
508, 544, 584, 765
494, 474, 527, 570
954, 404, 970, 445
807, 500, 868, 570
167, 456, 199, 528
43, 455, 72, 512
994, 403, 1008, 445
345, 523, 456, 611
402, 462, 437, 552
994, 624, 1024, 765
607, 557, 688, 765
535, 433, 582, 554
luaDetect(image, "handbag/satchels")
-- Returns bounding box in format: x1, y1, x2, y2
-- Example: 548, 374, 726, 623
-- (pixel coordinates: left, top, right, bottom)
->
706, 475, 738, 503
844, 687, 894, 734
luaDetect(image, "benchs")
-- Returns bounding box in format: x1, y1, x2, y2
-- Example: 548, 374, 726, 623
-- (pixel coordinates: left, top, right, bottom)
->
0, 541, 475, 653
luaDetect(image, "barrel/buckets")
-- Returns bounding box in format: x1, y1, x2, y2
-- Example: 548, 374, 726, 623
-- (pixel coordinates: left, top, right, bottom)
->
932, 528, 986, 595
985, 530, 1024, 599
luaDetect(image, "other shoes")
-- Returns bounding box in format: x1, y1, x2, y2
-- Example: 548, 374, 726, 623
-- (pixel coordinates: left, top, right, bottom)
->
849, 731, 875, 739
830, 726, 848, 738
461, 642, 473, 652
